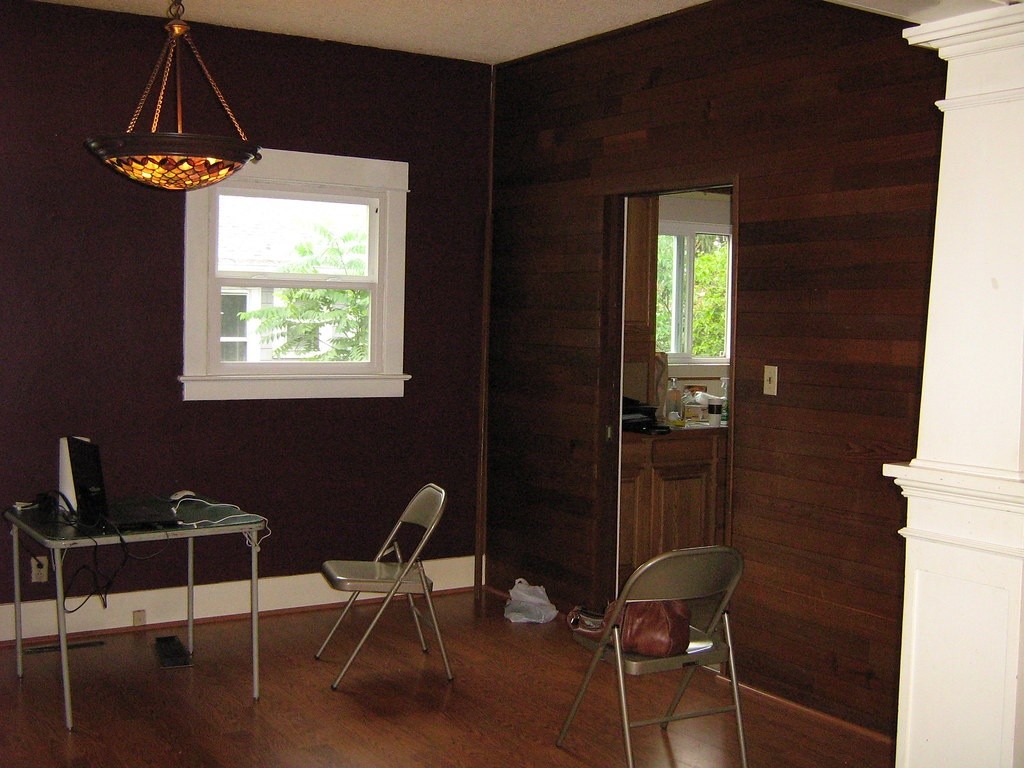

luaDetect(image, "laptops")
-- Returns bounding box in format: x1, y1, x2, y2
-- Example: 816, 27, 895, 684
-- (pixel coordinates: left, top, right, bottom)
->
66, 436, 183, 531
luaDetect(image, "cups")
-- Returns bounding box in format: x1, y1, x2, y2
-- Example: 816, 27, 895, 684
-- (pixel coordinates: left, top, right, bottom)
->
707, 398, 723, 428
669, 411, 678, 422
690, 407, 703, 422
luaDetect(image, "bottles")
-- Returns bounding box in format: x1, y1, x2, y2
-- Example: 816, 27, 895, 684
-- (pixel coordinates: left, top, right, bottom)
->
665, 377, 682, 425
719, 376, 730, 427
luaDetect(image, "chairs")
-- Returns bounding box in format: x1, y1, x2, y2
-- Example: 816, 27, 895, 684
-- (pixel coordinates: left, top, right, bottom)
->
556, 546, 749, 768
314, 483, 452, 690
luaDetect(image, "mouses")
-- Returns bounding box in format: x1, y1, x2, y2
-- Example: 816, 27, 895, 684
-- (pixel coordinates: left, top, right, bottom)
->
169, 490, 195, 504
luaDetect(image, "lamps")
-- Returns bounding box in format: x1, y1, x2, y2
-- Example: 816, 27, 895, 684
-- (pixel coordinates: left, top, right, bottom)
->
82, 0, 262, 192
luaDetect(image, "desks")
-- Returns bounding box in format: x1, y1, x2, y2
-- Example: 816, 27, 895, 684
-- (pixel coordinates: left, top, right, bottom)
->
5, 490, 267, 731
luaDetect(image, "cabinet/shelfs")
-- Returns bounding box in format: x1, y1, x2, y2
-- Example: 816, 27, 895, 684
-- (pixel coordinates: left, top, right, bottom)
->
624, 195, 659, 406
617, 420, 730, 601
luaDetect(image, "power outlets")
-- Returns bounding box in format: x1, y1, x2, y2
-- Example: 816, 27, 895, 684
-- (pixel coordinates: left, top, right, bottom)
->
31, 556, 48, 583
133, 609, 146, 626
763, 365, 778, 396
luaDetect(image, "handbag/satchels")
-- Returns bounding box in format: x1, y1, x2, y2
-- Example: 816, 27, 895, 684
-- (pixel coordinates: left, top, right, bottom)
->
567, 597, 690, 657
